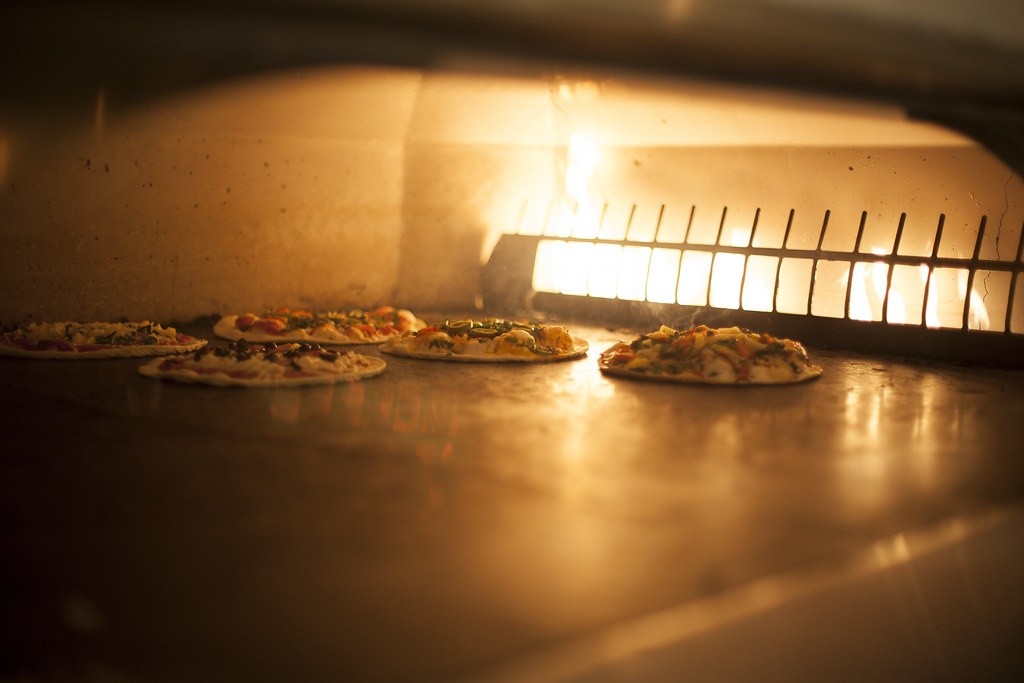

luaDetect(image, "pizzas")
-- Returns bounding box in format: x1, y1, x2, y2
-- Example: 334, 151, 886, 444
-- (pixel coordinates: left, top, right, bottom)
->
380, 319, 589, 361
600, 324, 822, 387
215, 307, 425, 345
0, 322, 208, 360
139, 336, 386, 386
